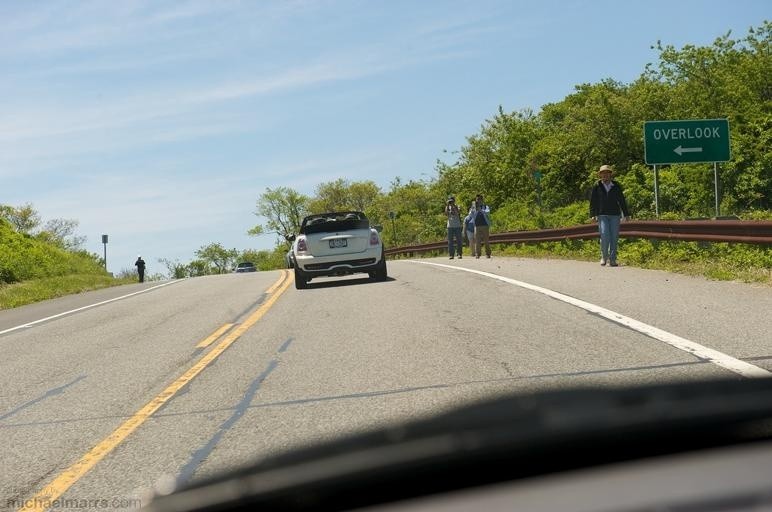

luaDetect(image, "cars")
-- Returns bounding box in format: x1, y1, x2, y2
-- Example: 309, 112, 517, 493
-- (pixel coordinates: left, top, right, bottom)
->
283, 244, 295, 268
285, 209, 388, 288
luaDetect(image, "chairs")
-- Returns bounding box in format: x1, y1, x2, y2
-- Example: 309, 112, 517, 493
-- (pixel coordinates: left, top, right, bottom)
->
311, 213, 358, 225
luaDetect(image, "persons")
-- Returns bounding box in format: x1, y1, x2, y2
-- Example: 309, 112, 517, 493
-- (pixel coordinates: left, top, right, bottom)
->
445, 197, 463, 259
135, 256, 146, 282
469, 194, 491, 259
462, 207, 482, 256
590, 165, 631, 266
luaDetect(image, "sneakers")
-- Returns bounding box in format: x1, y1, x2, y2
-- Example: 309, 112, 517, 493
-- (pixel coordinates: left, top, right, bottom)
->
610, 261, 618, 266
601, 258, 606, 265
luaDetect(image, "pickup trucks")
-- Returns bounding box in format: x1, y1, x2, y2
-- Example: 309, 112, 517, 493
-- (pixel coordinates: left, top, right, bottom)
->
232, 262, 256, 273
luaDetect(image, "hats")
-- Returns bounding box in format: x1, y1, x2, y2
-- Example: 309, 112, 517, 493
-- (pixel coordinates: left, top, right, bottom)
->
597, 165, 613, 174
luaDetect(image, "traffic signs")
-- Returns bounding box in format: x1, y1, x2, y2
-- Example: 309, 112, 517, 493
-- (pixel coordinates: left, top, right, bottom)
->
642, 119, 731, 164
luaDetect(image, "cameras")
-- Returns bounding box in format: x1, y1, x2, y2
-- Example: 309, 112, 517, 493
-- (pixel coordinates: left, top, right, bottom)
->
448, 200, 455, 204
